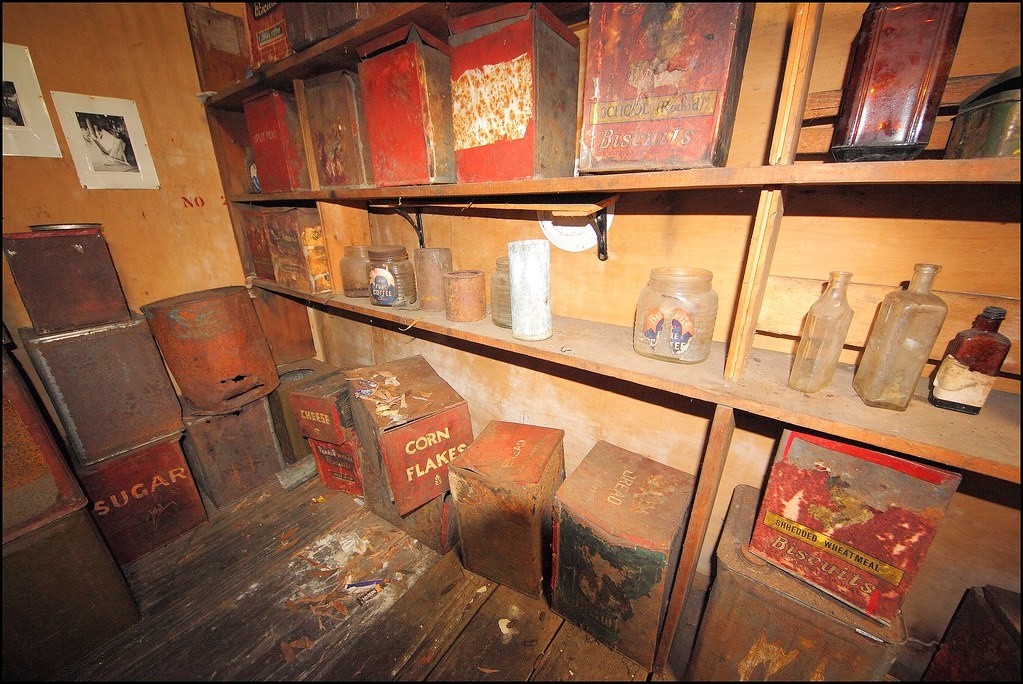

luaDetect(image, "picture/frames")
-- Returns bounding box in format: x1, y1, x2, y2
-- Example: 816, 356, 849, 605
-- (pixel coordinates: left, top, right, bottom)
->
2, 42, 62, 159
50, 90, 161, 191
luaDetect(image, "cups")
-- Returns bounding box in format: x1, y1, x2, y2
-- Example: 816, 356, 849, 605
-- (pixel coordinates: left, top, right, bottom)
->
413, 248, 452, 312
508, 240, 553, 341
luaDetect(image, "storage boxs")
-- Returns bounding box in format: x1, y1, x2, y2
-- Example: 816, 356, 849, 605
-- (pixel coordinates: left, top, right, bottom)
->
241, 1, 756, 297
290, 353, 961, 684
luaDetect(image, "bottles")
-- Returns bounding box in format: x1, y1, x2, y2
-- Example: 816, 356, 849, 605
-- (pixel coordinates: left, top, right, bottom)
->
788, 271, 854, 393
339, 246, 369, 298
930, 307, 1012, 415
634, 268, 718, 365
490, 256, 512, 329
853, 263, 949, 412
367, 245, 415, 307
